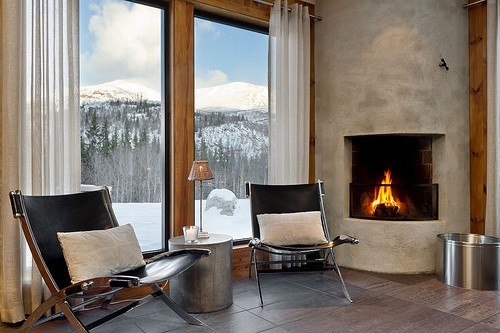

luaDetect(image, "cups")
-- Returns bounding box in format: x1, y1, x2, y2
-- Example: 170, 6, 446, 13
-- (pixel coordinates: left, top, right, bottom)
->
182, 226, 199, 243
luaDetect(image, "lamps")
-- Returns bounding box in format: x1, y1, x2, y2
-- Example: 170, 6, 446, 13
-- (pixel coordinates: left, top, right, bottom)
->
188, 160, 214, 239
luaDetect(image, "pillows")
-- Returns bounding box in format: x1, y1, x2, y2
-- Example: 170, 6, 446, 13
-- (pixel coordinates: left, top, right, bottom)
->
256, 211, 329, 246
57, 223, 146, 284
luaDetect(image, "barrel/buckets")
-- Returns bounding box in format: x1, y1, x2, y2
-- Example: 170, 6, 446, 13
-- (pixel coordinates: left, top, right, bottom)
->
435, 231, 500, 291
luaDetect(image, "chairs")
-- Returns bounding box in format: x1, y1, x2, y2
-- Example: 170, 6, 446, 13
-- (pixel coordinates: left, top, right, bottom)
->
9, 186, 212, 333
246, 179, 359, 307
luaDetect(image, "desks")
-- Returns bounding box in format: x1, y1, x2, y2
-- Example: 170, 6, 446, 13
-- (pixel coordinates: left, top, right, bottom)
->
168, 234, 233, 313
